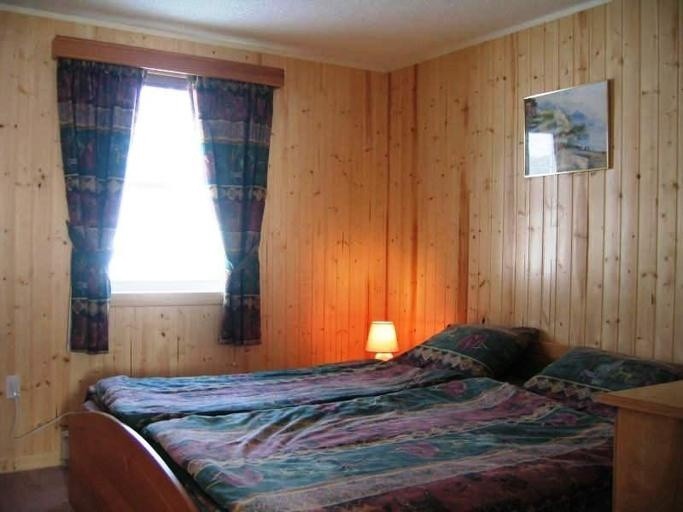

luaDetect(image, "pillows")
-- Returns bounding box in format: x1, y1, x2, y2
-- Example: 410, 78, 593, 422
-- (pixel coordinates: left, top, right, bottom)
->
387, 326, 681, 415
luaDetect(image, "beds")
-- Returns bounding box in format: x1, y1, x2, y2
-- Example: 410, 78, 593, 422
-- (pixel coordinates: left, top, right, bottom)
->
57, 321, 681, 509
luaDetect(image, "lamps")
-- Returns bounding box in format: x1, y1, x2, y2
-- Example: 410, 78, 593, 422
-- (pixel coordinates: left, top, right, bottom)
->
362, 316, 397, 365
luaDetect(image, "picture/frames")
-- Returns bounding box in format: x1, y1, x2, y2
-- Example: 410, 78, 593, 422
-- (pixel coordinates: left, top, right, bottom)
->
517, 80, 615, 179
